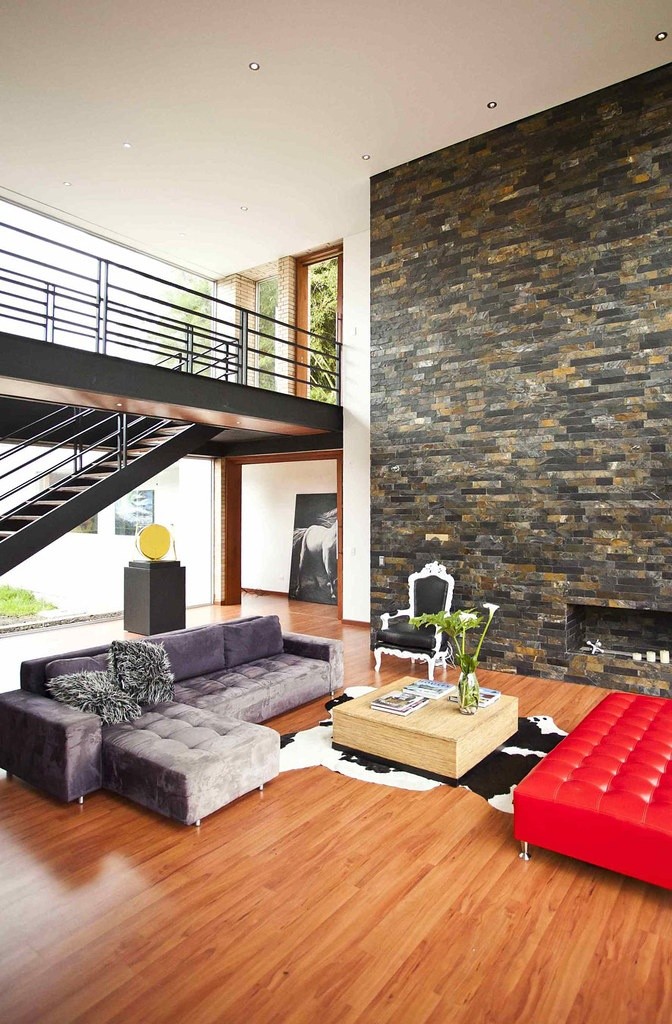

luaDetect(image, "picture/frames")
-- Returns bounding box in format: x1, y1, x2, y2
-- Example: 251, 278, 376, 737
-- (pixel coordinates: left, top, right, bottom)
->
114, 490, 155, 536
70, 513, 98, 534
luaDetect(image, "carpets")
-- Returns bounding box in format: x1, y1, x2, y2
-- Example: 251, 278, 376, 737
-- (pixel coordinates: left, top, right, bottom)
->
280, 685, 569, 814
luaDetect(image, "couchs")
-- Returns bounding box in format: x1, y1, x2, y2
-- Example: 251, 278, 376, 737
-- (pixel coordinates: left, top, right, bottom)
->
514, 692, 672, 891
0, 614, 344, 827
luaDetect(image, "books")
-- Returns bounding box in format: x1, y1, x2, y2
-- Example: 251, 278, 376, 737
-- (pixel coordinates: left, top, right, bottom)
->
403, 678, 457, 700
370, 690, 430, 717
449, 685, 501, 708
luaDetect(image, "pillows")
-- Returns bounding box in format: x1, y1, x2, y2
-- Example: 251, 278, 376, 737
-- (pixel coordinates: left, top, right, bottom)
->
108, 639, 175, 704
93, 653, 109, 672
222, 614, 284, 670
44, 657, 102, 683
150, 625, 225, 683
45, 671, 141, 725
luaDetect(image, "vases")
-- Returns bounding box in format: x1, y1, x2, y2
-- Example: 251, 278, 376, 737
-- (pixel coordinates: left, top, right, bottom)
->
456, 672, 480, 716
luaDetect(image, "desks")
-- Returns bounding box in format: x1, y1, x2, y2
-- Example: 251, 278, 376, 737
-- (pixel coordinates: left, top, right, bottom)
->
123, 560, 186, 636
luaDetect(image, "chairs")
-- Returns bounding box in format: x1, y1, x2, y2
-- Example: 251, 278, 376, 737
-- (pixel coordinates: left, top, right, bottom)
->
373, 560, 455, 681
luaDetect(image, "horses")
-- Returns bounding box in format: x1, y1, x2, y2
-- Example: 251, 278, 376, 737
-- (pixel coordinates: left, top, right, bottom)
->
294, 507, 338, 600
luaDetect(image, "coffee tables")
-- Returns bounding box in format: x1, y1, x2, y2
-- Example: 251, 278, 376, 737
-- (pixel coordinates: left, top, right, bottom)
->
332, 676, 519, 779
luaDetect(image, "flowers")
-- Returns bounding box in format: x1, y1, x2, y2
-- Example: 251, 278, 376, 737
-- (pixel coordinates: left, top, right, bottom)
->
409, 602, 500, 714
